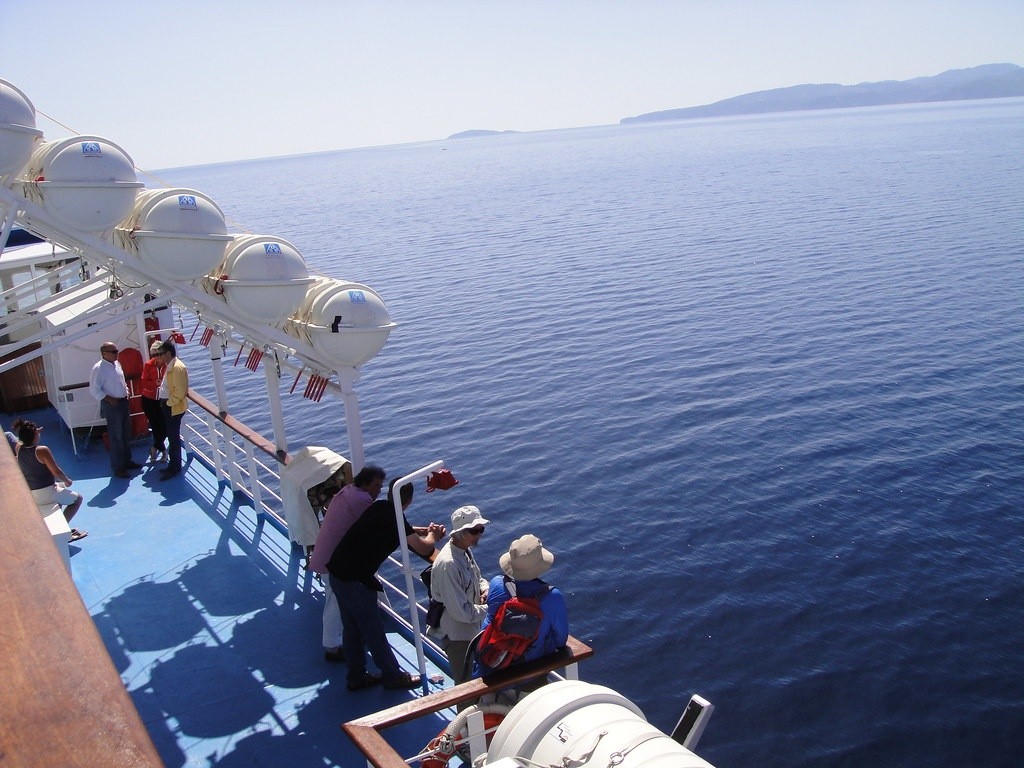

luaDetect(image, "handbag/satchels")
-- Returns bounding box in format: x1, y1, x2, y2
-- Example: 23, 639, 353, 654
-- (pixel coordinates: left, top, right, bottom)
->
426, 600, 445, 627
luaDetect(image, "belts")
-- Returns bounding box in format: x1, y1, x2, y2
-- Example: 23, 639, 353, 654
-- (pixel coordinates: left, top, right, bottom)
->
116, 396, 127, 401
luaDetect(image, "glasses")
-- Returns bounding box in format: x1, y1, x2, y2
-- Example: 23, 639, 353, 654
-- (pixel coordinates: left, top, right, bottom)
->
466, 527, 484, 533
105, 350, 118, 354
160, 352, 165, 356
152, 353, 159, 357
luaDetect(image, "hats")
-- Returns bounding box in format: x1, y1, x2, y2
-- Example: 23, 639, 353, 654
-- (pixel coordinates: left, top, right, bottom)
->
499, 533, 555, 581
449, 506, 490, 534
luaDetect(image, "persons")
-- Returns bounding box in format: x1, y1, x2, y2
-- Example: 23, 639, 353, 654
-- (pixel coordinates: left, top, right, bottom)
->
325, 475, 447, 692
89, 341, 143, 481
309, 465, 388, 660
4, 416, 84, 524
141, 339, 189, 481
430, 502, 570, 762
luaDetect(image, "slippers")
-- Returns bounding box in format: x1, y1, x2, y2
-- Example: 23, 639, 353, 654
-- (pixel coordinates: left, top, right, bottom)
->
68, 528, 86, 544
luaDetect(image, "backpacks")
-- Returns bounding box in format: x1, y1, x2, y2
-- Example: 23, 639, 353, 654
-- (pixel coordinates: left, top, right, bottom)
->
469, 576, 554, 677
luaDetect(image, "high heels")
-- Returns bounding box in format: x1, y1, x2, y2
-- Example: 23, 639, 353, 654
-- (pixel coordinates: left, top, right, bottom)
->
148, 447, 156, 464
160, 450, 168, 460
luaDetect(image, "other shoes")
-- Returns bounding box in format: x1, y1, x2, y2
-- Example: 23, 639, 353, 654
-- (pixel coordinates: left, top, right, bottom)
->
124, 459, 137, 466
324, 652, 337, 661
346, 669, 376, 691
391, 671, 422, 690
116, 469, 133, 477
158, 461, 177, 480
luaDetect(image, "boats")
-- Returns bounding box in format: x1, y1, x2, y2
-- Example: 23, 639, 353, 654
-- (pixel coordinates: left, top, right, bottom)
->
0, 77, 717, 768
